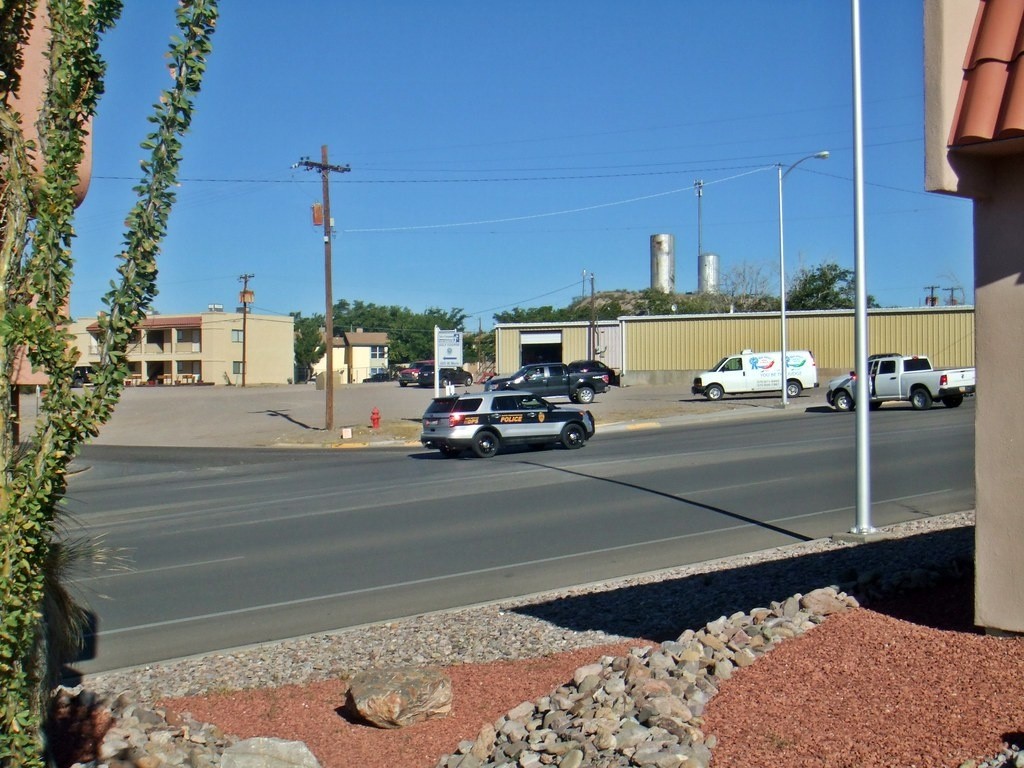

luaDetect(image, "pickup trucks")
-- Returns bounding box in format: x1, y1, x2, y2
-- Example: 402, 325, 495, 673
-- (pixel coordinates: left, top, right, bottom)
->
484, 362, 609, 405
826, 352, 976, 412
70, 366, 98, 388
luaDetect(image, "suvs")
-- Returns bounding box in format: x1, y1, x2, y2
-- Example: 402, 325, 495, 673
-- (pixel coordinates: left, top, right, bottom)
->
419, 364, 473, 388
420, 391, 596, 458
398, 360, 435, 387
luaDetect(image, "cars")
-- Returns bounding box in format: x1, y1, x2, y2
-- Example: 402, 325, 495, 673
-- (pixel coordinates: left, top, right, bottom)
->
567, 360, 615, 385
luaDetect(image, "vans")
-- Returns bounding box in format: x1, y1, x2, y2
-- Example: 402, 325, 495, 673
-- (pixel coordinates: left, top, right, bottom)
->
691, 349, 820, 401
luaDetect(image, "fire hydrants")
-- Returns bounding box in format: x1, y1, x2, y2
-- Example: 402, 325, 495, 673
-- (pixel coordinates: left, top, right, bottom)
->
370, 406, 382, 429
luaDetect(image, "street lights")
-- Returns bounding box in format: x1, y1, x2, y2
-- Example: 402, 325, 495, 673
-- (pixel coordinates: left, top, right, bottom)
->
778, 151, 830, 405
